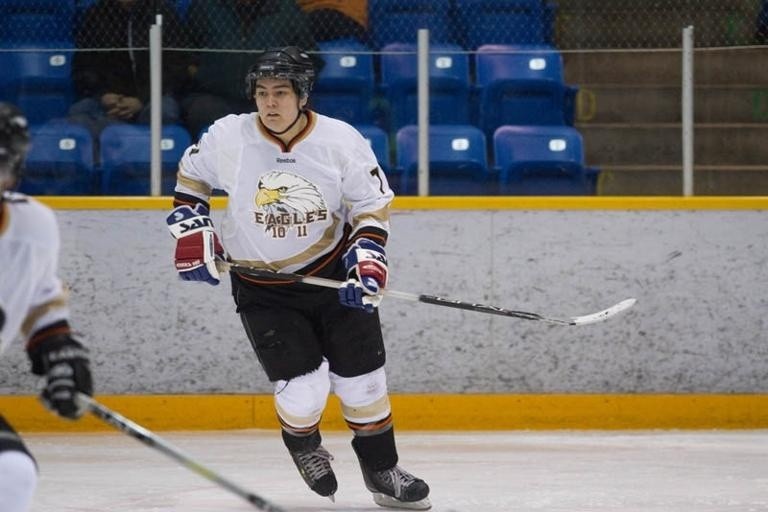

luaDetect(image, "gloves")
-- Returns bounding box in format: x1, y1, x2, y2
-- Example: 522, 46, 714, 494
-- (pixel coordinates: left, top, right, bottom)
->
337, 237, 388, 313
24, 319, 93, 420
165, 202, 229, 286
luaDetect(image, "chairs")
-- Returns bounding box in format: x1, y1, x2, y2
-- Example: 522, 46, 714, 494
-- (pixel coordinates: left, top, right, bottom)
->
0, 0, 602, 198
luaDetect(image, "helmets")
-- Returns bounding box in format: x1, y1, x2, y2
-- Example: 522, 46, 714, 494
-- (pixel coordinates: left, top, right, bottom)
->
0, 100, 31, 196
244, 44, 316, 99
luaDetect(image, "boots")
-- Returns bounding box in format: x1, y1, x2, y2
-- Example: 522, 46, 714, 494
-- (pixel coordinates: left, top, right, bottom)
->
350, 424, 430, 502
280, 426, 338, 497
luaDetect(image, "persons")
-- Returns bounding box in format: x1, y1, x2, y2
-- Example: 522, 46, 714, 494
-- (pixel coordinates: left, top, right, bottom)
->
165, 47, 431, 504
298, 0, 370, 44
0, 98, 94, 509
180, 1, 328, 138
67, 0, 185, 125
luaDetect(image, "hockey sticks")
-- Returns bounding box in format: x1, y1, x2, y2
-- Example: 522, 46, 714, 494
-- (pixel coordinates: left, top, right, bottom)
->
215, 258, 637, 326
